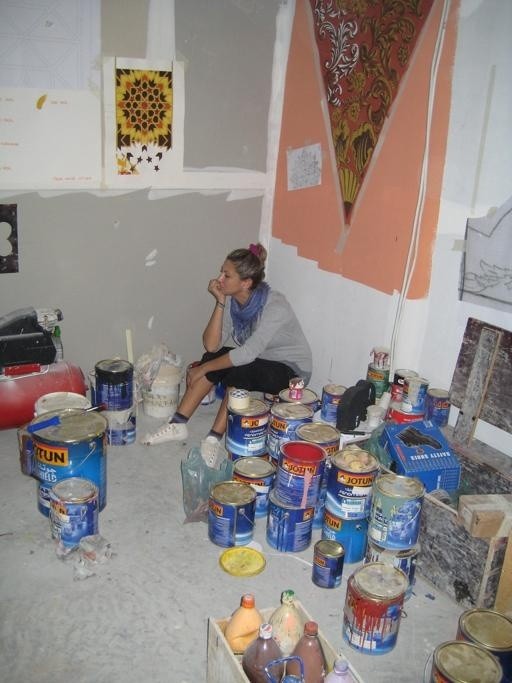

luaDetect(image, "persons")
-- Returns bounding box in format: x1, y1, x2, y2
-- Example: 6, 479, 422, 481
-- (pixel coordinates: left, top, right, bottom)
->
140, 242, 312, 469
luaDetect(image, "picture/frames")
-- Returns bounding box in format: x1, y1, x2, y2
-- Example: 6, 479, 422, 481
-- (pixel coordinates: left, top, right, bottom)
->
102, 55, 186, 190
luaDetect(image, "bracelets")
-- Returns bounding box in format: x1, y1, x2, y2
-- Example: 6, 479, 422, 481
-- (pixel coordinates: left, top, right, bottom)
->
217, 301, 225, 309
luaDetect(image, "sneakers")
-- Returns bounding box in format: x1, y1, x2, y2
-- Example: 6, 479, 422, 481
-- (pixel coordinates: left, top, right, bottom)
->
139, 416, 188, 445
200, 434, 224, 467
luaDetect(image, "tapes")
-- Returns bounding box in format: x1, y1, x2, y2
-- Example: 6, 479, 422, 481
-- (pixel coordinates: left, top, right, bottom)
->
228, 388, 250, 410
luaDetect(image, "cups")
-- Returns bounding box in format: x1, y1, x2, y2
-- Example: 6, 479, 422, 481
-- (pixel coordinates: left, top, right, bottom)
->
366, 404, 384, 426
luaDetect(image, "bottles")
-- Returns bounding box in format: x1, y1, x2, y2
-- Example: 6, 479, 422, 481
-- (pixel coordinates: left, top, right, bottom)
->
287, 620, 328, 683
225, 591, 267, 655
322, 658, 354, 683
241, 623, 284, 683
271, 588, 309, 656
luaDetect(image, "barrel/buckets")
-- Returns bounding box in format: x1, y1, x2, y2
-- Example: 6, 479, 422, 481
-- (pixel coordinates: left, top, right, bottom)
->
28, 348, 512, 683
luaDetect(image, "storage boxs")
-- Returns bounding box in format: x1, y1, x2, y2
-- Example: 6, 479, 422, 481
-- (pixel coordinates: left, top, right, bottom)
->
204, 596, 366, 683
343, 432, 511, 610
380, 419, 462, 492
